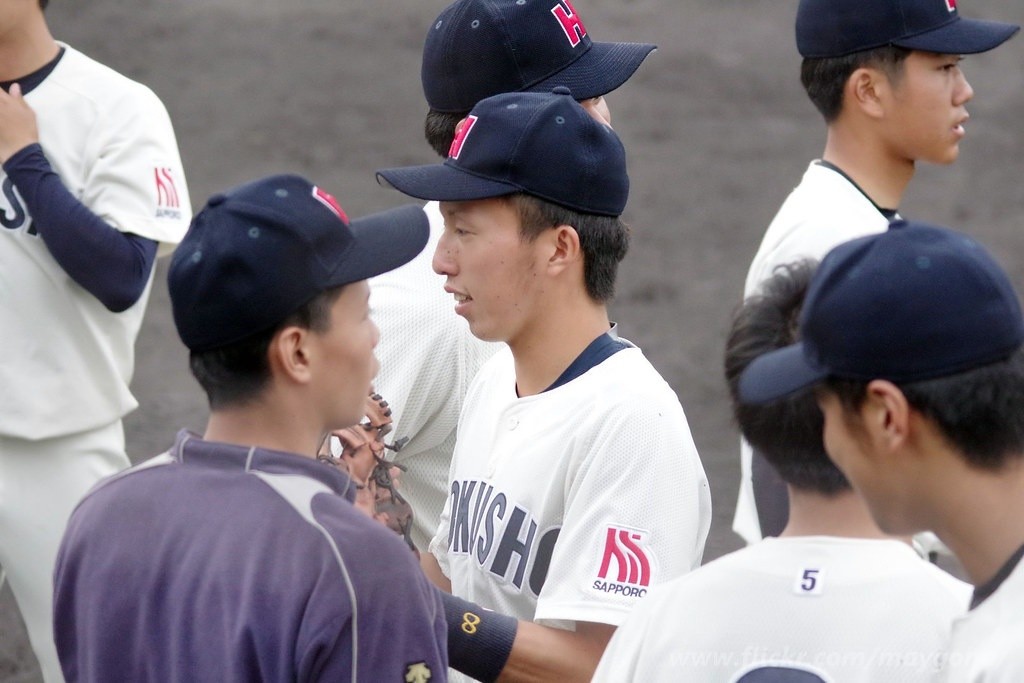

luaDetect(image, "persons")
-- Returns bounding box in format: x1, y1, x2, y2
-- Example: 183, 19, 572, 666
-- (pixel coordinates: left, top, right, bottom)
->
316, 86, 713, 683
52, 175, 447, 683
740, 217, 1023, 683
1, 1, 195, 683
729, 0, 1020, 562
330, 0, 661, 550
588, 260, 976, 683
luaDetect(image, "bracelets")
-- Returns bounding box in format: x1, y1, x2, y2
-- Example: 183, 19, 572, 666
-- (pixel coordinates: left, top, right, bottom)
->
440, 589, 518, 683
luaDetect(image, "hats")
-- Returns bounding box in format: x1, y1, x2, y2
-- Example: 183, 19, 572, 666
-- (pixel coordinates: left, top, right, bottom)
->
421, 0, 658, 112
375, 85, 629, 216
168, 175, 431, 350
738, 219, 1024, 403
796, 0, 1020, 59
